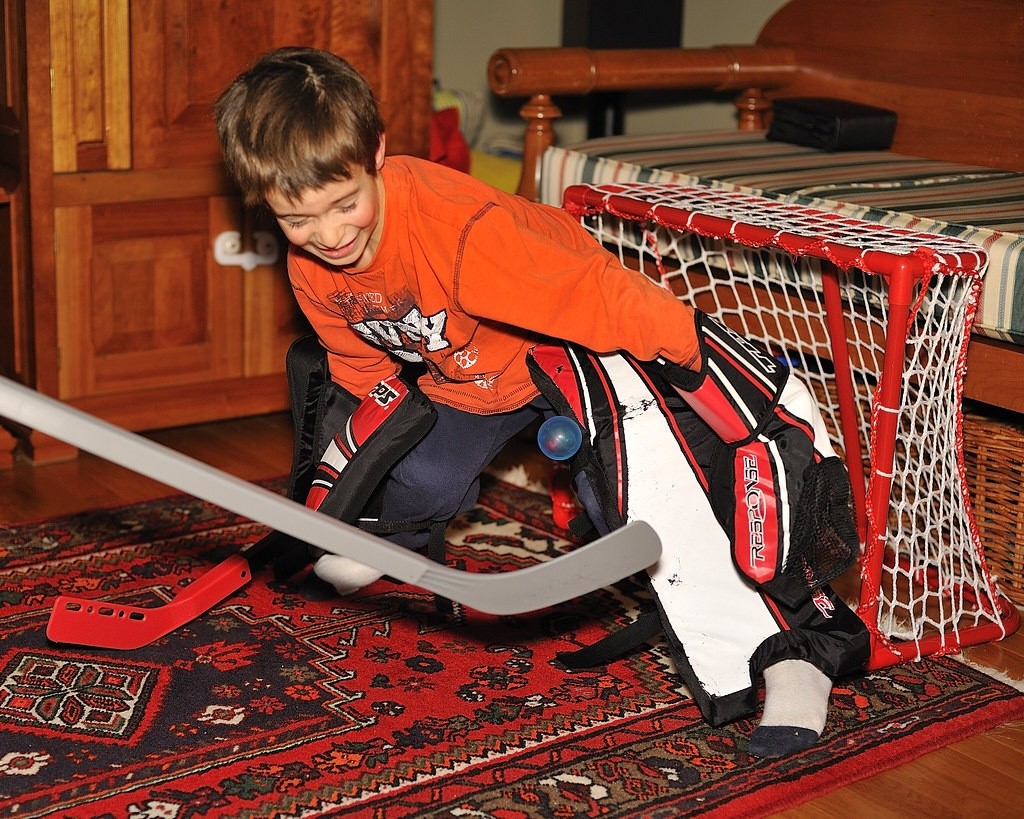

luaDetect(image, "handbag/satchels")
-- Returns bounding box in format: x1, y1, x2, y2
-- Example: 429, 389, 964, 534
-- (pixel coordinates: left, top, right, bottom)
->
765, 95, 898, 152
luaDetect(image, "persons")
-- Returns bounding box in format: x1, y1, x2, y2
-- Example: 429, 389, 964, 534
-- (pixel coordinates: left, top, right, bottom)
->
213, 46, 853, 750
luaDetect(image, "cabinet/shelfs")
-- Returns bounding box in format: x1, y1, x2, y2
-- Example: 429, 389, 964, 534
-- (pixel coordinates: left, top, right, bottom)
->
0, 0, 439, 466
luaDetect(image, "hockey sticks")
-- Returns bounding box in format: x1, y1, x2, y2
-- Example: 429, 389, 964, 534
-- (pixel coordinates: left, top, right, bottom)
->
0, 367, 665, 616
44, 527, 290, 652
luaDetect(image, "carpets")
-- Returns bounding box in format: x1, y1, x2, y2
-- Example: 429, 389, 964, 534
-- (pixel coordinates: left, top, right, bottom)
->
0, 454, 1024, 819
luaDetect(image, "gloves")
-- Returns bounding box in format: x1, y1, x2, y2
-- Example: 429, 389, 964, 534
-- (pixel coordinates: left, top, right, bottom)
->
269, 372, 437, 579
656, 307, 855, 584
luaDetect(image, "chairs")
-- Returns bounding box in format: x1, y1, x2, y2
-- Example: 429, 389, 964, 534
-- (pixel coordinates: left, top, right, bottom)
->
488, 1, 1024, 417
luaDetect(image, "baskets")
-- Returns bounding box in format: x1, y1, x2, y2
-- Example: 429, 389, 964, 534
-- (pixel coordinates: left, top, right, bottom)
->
791, 365, 1024, 603
